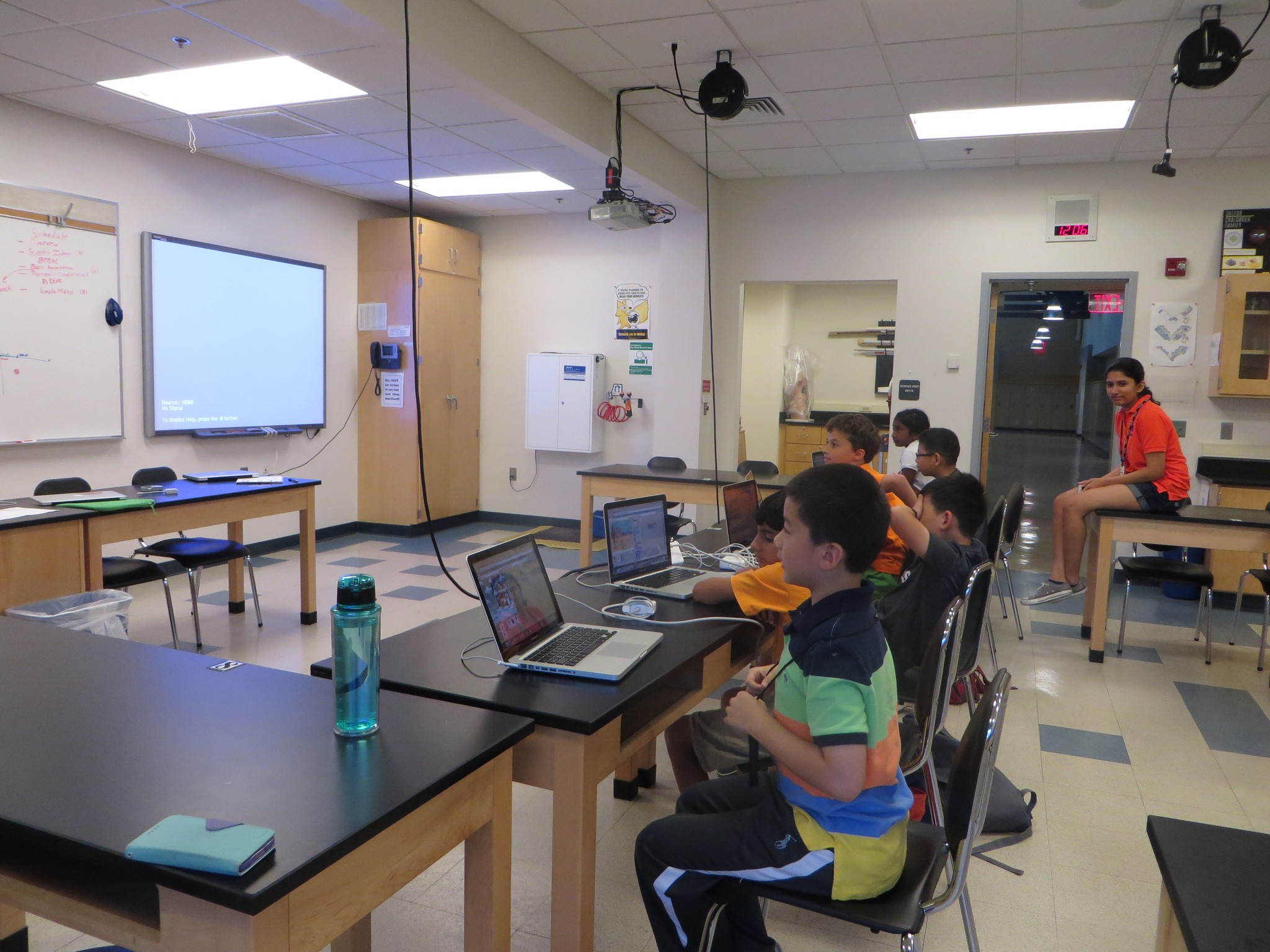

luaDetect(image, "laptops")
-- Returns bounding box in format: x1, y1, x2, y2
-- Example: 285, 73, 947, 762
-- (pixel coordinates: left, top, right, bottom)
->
30, 491, 127, 506
182, 471, 253, 481
722, 478, 759, 558
812, 451, 825, 466
466, 532, 663, 684
604, 493, 735, 600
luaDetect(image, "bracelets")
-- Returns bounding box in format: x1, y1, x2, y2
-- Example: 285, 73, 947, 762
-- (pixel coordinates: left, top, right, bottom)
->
910, 507, 918, 518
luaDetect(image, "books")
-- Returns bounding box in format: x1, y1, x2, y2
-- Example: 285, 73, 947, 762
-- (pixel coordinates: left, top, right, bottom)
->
235, 475, 284, 484
124, 814, 278, 876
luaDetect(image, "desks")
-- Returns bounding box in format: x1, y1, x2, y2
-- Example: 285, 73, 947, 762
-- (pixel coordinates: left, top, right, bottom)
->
1081, 503, 1270, 665
0, 448, 827, 952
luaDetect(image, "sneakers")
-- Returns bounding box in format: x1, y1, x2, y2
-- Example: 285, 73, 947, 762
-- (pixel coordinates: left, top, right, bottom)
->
1019, 578, 1075, 605
1052, 581, 1087, 604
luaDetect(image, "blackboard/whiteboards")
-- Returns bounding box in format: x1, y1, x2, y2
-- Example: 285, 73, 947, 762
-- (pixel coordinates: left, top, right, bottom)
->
0, 204, 127, 445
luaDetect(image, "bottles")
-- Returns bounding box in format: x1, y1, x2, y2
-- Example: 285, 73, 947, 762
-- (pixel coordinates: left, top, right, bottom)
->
330, 572, 382, 737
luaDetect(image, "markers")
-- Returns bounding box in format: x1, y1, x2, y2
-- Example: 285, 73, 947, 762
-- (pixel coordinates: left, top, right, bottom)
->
214, 431, 225, 434
16, 439, 37, 443
231, 430, 244, 433
276, 429, 289, 431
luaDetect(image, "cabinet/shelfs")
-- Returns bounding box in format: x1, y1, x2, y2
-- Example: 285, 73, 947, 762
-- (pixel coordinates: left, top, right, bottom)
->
358, 216, 481, 526
524, 353, 606, 454
1207, 273, 1270, 398
779, 422, 888, 474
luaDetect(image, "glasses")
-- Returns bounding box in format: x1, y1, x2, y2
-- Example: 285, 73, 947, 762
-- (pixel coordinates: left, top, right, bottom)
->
915, 452, 938, 460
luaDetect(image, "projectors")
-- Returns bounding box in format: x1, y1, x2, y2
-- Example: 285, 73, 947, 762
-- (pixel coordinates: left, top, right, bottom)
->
590, 201, 651, 232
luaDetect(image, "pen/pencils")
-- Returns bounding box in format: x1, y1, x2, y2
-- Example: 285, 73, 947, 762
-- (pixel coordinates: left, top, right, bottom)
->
288, 478, 299, 482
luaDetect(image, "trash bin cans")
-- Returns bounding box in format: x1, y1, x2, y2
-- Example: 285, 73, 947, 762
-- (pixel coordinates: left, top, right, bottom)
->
4, 587, 133, 641
1159, 546, 1207, 600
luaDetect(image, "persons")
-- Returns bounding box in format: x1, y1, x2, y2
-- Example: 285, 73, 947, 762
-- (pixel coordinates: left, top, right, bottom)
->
634, 408, 989, 794
1020, 357, 1192, 606
634, 462, 913, 952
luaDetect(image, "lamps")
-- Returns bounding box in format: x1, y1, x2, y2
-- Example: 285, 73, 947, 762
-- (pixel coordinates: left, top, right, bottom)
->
1030, 323, 1052, 349
1043, 292, 1064, 320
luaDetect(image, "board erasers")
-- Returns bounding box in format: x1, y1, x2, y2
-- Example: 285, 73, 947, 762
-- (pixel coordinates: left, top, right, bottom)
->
245, 428, 262, 432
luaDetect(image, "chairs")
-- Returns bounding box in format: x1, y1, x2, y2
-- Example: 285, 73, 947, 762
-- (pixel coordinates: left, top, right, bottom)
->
996, 479, 1026, 639
878, 595, 989, 888
696, 668, 1023, 952
643, 456, 698, 541
130, 466, 263, 648
1110, 542, 1212, 665
34, 476, 182, 650
1228, 500, 1270, 671
986, 495, 1009, 673
953, 562, 994, 715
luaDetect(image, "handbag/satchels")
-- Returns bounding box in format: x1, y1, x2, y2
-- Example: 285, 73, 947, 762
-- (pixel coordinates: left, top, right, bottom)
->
949, 664, 988, 706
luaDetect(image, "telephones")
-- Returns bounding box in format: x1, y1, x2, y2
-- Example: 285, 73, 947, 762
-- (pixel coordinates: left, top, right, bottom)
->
370, 342, 402, 369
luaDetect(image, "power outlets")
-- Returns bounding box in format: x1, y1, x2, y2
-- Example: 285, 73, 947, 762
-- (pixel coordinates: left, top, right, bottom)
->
509, 467, 517, 481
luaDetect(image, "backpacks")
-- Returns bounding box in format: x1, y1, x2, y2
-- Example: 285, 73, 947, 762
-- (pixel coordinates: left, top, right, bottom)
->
917, 733, 1037, 842
